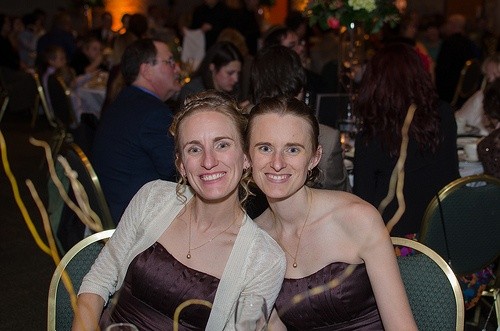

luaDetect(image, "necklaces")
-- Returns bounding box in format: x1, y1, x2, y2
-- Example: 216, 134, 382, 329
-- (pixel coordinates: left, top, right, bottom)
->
187, 199, 241, 259
271, 187, 313, 268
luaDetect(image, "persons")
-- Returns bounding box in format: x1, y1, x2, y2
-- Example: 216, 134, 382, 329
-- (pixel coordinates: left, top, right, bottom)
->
71, 89, 287, 331
242, 92, 419, 331
0, 0, 500, 238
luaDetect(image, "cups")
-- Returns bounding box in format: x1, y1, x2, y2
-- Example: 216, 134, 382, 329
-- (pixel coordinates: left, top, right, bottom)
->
464, 144, 480, 162
235, 290, 268, 331
104, 323, 139, 331
302, 91, 353, 160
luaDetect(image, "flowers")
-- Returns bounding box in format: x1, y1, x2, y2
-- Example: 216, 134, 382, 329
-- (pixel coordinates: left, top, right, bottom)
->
302, 0, 403, 35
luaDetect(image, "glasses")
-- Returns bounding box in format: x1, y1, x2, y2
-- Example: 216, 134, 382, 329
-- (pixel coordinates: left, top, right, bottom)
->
142, 57, 178, 69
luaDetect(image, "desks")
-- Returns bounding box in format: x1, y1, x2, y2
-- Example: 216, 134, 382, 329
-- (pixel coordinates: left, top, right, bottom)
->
71, 73, 107, 120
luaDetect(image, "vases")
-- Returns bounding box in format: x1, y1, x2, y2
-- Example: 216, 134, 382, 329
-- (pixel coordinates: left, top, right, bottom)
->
336, 24, 365, 79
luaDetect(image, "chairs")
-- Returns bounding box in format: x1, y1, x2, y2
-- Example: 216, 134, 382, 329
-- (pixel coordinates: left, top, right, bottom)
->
0, 66, 116, 331
419, 174, 500, 331
450, 59, 485, 109
391, 237, 464, 331
